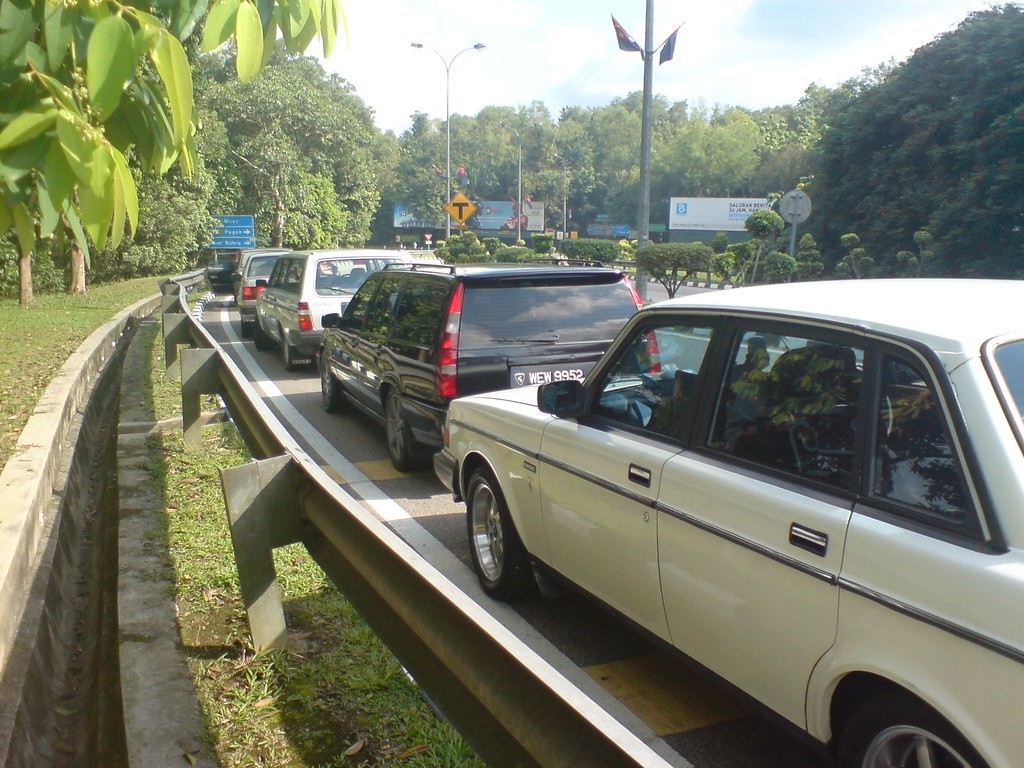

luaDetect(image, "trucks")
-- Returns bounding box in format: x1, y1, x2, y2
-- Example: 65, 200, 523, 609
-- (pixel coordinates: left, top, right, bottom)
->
585, 223, 631, 239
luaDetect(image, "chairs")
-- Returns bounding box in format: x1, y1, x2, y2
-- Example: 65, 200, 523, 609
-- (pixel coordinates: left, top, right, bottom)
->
769, 345, 856, 468
672, 364, 765, 454
349, 268, 365, 287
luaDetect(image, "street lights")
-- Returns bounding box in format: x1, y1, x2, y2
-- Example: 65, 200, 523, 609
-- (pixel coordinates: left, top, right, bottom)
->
408, 40, 486, 248
498, 119, 543, 248
551, 152, 568, 238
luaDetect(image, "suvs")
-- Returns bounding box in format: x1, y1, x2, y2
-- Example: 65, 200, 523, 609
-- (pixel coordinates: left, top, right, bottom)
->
314, 258, 665, 472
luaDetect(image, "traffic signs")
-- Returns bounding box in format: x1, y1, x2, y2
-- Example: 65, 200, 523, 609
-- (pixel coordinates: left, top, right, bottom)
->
202, 215, 255, 248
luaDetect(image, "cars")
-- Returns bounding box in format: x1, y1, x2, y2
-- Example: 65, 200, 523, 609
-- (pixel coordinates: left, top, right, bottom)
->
252, 249, 416, 373
432, 276, 1024, 768
231, 247, 295, 338
